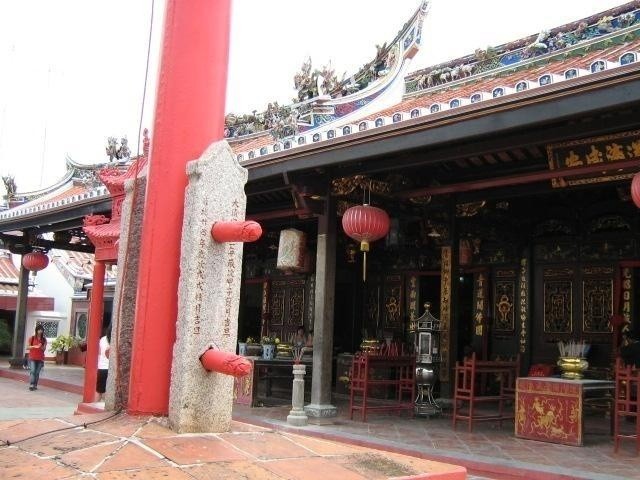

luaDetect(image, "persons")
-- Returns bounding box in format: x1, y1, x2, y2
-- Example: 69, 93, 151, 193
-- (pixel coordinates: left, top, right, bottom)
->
289, 326, 314, 347
25, 324, 47, 391
96, 322, 113, 402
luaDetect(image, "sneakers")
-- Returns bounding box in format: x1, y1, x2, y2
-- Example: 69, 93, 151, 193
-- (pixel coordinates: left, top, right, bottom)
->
29, 384, 37, 391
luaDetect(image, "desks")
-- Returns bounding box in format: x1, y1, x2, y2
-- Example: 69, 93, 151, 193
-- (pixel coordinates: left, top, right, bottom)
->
231, 356, 314, 409
515, 376, 616, 447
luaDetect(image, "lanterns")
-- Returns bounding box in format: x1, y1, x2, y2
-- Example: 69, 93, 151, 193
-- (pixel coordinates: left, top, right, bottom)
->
631, 173, 640, 209
341, 205, 391, 242
22, 252, 49, 273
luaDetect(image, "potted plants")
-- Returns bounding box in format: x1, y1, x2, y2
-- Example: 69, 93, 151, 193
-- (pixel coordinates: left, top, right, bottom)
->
48, 335, 82, 364
